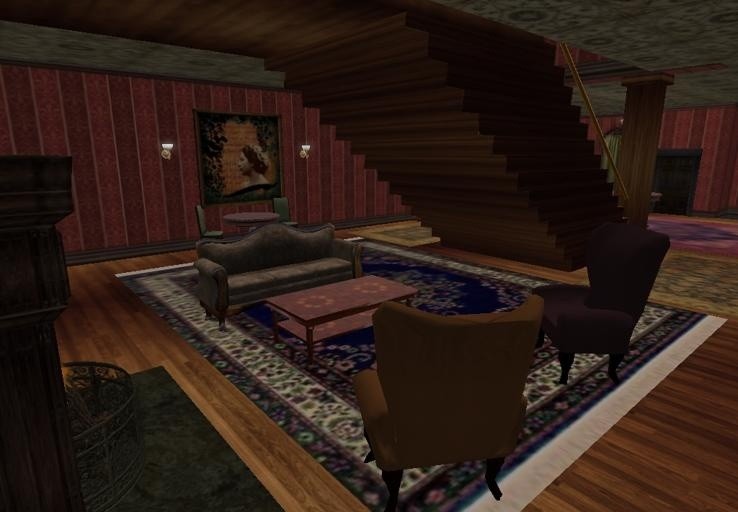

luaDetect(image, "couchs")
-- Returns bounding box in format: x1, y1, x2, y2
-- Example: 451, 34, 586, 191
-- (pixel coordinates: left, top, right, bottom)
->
193, 222, 365, 330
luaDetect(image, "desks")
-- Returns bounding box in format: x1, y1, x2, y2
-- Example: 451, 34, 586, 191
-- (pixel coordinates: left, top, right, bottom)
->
223, 212, 280, 240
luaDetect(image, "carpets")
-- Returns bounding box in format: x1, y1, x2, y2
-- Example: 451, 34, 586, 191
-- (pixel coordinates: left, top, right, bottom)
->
648, 217, 738, 258
113, 233, 728, 512
70, 366, 283, 512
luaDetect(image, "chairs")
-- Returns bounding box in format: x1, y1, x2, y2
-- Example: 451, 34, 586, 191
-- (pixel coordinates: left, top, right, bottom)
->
356, 294, 544, 512
273, 197, 298, 227
195, 204, 224, 239
533, 223, 670, 386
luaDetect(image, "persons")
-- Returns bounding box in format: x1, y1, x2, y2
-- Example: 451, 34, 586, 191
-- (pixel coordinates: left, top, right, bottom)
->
238, 144, 269, 188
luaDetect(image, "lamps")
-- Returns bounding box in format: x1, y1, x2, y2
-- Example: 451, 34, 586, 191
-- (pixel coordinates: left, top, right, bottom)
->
297, 144, 311, 160
160, 143, 174, 161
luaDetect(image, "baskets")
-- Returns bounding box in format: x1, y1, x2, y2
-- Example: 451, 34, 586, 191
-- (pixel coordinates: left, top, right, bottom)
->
62, 362, 144, 511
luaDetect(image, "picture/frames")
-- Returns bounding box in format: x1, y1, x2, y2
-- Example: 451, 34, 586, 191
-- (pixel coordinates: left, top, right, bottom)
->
191, 109, 284, 208
